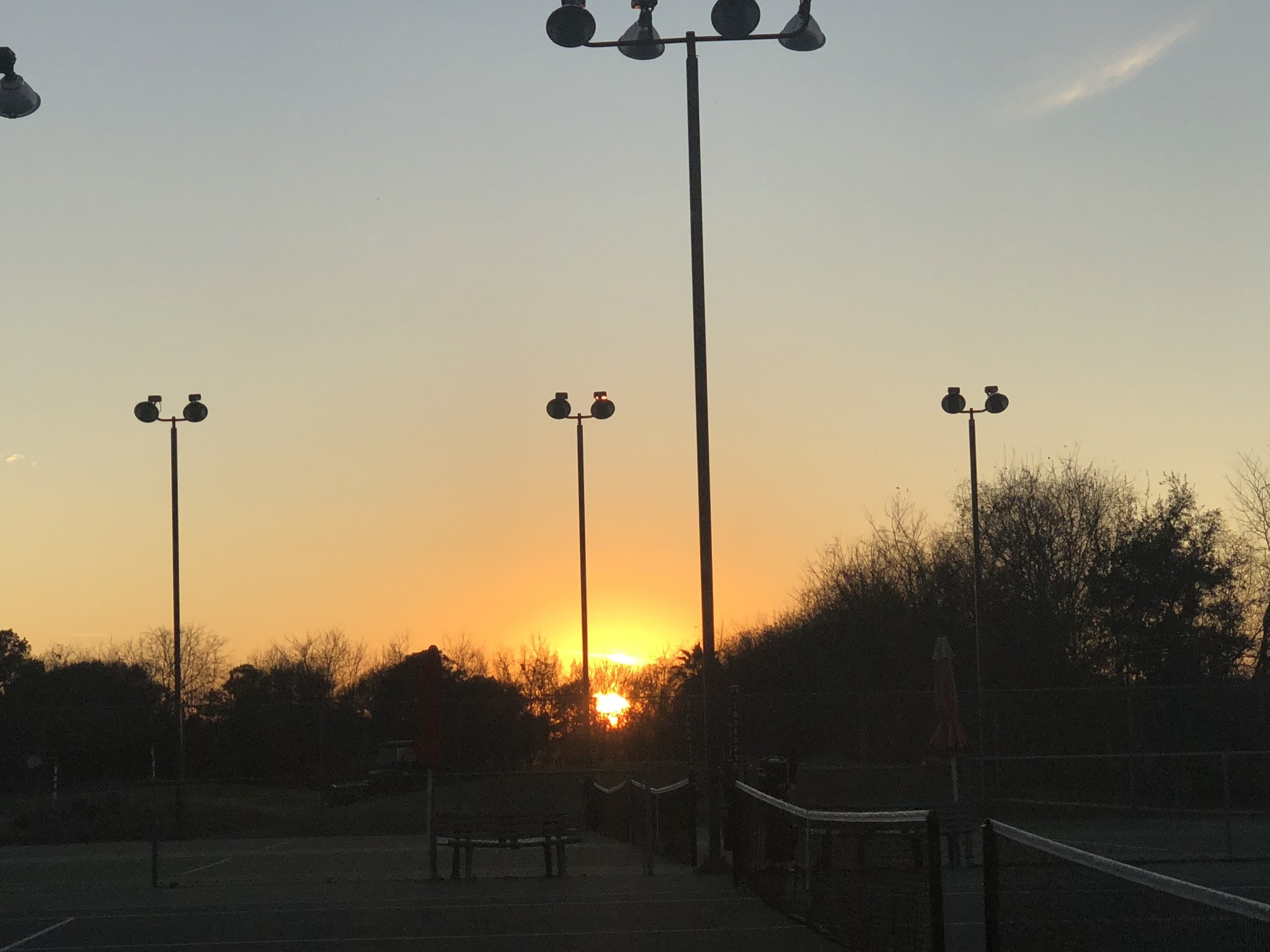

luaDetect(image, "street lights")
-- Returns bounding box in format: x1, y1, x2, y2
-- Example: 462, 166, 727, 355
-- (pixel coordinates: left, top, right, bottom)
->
937, 385, 1010, 815
546, 390, 616, 747
543, 0, 824, 882
133, 391, 208, 803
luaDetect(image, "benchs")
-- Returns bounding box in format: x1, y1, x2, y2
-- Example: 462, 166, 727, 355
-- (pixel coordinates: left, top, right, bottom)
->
426, 812, 577, 881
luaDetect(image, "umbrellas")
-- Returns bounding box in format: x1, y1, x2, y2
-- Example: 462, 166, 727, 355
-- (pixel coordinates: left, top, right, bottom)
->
928, 638, 971, 805
411, 648, 445, 847
